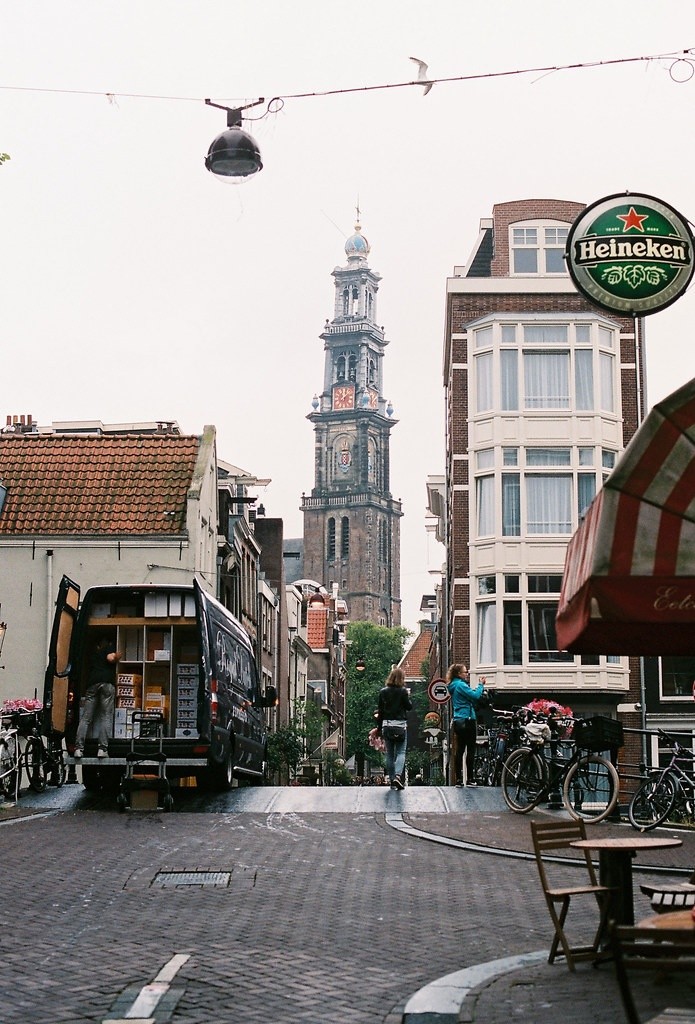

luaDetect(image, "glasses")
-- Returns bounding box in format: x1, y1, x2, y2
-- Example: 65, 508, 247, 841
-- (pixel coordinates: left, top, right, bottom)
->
459, 670, 468, 674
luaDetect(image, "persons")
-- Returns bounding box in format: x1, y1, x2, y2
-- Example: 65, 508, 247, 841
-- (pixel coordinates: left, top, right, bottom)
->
74, 634, 124, 758
376, 669, 413, 789
445, 663, 485, 788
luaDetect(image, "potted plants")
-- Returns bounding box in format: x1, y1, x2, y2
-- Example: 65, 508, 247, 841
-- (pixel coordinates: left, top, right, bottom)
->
291, 696, 328, 782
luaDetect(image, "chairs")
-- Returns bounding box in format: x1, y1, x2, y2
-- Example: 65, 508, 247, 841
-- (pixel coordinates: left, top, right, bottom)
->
530, 816, 620, 974
608, 867, 695, 1024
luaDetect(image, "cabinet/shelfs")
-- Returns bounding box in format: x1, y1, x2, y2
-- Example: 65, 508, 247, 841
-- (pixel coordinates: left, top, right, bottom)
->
86, 613, 199, 738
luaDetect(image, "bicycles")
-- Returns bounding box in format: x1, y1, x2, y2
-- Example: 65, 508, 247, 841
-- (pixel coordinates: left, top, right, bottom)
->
0, 706, 68, 800
627, 728, 695, 832
475, 703, 623, 824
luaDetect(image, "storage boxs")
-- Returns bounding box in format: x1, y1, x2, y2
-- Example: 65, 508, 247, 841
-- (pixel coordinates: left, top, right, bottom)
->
78, 589, 203, 740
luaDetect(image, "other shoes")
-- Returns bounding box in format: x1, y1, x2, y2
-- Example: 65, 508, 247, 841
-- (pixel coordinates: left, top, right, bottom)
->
98, 750, 109, 758
391, 785, 398, 791
74, 750, 83, 758
394, 778, 405, 790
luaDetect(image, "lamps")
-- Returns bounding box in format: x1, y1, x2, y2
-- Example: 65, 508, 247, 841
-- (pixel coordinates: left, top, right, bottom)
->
204, 96, 264, 177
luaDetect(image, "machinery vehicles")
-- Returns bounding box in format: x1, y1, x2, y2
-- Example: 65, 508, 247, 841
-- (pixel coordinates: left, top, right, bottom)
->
44, 573, 277, 796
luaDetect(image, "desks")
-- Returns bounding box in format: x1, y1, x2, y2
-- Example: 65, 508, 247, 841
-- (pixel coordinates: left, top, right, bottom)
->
567, 837, 686, 970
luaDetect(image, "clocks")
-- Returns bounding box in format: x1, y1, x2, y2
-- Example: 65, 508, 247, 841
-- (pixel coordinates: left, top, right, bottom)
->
331, 384, 355, 411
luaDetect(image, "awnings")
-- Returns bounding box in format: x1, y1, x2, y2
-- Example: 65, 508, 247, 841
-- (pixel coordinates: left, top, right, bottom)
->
554, 376, 695, 656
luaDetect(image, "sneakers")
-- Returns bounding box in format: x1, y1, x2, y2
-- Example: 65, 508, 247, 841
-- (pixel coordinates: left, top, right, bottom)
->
466, 780, 484, 787
455, 781, 465, 788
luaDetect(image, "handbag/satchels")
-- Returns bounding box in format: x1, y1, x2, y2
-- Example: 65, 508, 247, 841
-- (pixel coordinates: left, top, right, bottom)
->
454, 718, 470, 735
385, 727, 406, 742
